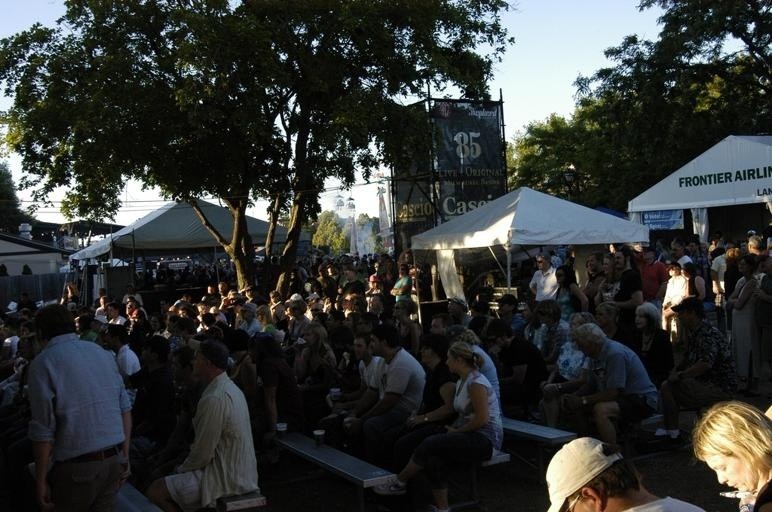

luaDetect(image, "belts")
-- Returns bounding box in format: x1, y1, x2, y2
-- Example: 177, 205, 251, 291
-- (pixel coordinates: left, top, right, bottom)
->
66, 442, 125, 464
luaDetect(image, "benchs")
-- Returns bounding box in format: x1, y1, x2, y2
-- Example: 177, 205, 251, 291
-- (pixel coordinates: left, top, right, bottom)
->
503, 416, 577, 489
272, 426, 396, 512
467, 450, 510, 497
219, 491, 269, 510
637, 412, 667, 443
110, 480, 162, 512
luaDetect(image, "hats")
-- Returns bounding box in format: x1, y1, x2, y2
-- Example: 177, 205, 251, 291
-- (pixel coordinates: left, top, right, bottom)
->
448, 296, 467, 312
286, 293, 302, 303
242, 303, 257, 312
671, 296, 704, 312
637, 303, 660, 319
496, 293, 518, 305
546, 436, 624, 512
132, 309, 145, 319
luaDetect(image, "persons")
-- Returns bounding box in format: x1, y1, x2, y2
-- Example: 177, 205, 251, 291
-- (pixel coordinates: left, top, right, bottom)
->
1, 232, 772, 511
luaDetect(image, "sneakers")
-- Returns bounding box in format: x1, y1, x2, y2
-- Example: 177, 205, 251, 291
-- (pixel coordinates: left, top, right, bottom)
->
374, 477, 407, 495
631, 430, 685, 454
738, 381, 762, 397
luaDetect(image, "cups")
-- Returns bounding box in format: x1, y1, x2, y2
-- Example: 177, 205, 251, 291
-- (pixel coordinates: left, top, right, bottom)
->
329, 388, 341, 402
312, 429, 326, 445
276, 422, 287, 437
